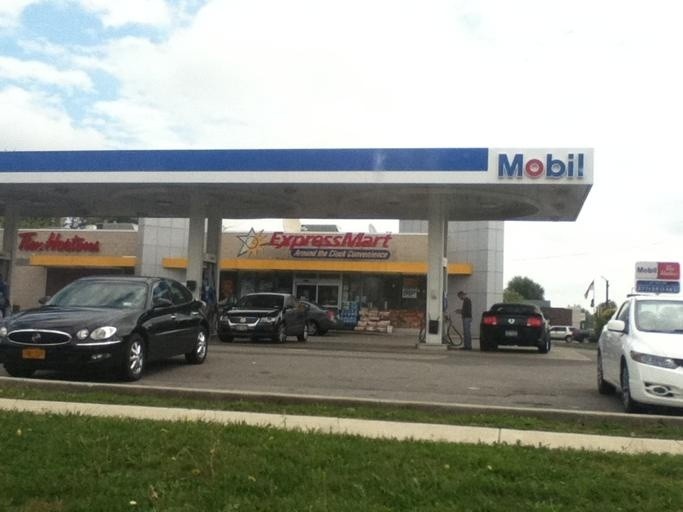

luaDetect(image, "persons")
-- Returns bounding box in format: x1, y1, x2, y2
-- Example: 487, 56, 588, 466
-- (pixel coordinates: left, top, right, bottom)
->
451, 290, 473, 350
217, 286, 238, 313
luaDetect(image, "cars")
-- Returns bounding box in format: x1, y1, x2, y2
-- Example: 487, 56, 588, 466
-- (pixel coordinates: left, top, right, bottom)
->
596, 296, 683, 413
298, 300, 336, 336
217, 292, 308, 343
0, 275, 209, 382
479, 303, 595, 353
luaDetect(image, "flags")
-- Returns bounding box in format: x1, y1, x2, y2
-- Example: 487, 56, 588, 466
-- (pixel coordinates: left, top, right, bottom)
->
583, 280, 594, 299
